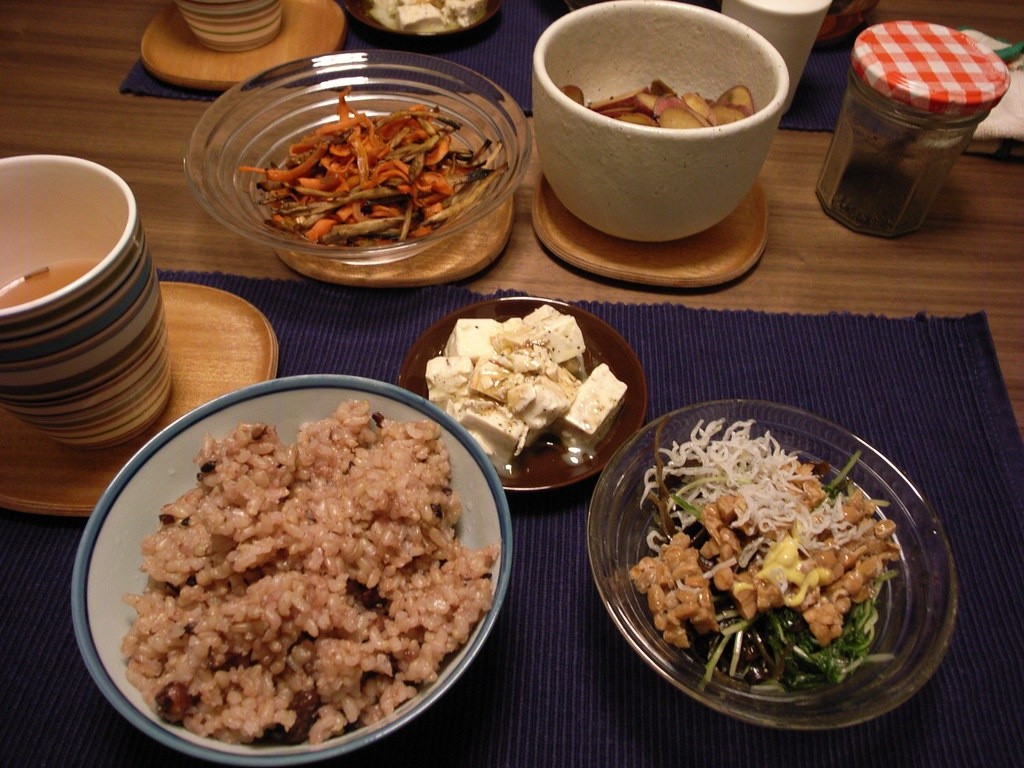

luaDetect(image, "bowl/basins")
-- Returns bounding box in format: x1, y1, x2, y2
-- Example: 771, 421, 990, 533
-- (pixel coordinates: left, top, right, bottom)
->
71, 372, 513, 767
586, 398, 958, 732
183, 48, 531, 266
531, 0, 790, 242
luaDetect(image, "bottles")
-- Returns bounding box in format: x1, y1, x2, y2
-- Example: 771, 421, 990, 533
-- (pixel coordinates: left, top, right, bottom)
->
813, 20, 1012, 239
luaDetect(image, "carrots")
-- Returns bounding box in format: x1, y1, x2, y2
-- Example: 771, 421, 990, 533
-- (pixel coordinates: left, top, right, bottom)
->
236, 85, 455, 243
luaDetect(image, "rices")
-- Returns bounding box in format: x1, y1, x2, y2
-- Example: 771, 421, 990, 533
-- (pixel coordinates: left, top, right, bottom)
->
120, 397, 502, 746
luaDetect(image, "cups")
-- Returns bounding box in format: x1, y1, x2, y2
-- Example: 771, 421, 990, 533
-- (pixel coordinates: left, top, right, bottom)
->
720, 0, 835, 114
0, 153, 171, 451
174, 0, 283, 53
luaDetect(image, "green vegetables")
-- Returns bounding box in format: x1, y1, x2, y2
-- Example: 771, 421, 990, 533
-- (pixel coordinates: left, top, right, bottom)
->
667, 447, 903, 692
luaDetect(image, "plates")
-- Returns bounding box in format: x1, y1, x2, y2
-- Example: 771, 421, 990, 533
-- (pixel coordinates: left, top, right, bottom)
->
394, 295, 649, 492
529, 154, 773, 289
343, 0, 504, 48
262, 198, 516, 284
2, 279, 280, 518
138, 2, 347, 91
